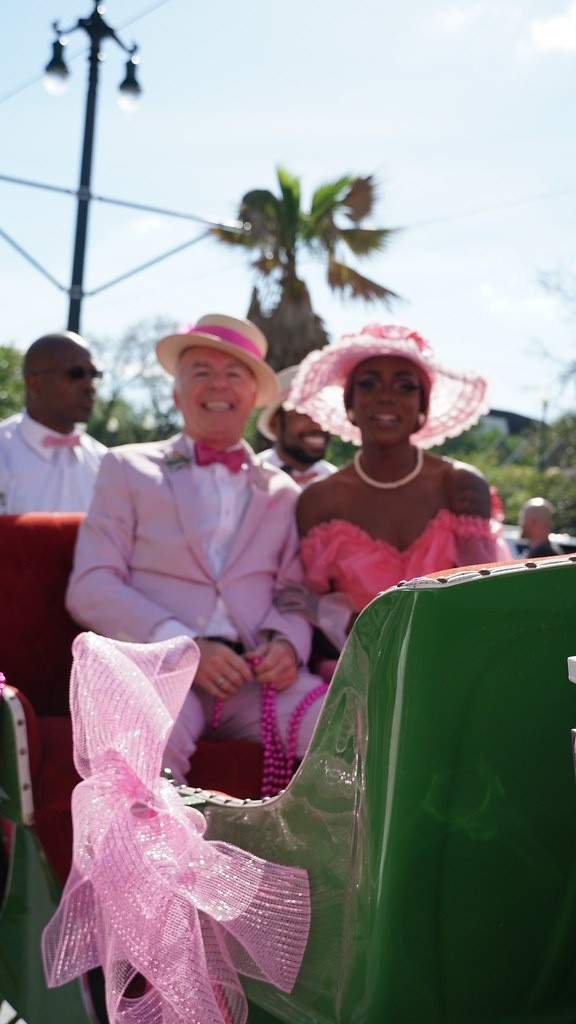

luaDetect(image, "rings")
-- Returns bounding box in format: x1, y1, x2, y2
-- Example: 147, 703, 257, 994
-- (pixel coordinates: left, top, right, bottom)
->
215, 677, 224, 685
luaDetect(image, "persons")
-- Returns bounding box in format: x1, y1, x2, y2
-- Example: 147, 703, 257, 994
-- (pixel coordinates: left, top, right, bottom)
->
60, 313, 330, 785
271, 324, 512, 677
251, 363, 340, 489
517, 495, 564, 560
1, 331, 109, 516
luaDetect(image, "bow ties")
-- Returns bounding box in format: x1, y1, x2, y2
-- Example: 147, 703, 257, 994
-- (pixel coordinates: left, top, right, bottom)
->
194, 439, 246, 473
43, 436, 81, 449
288, 471, 317, 483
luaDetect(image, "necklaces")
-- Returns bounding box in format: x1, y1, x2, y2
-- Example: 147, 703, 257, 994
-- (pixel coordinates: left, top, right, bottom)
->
354, 445, 424, 490
207, 654, 329, 799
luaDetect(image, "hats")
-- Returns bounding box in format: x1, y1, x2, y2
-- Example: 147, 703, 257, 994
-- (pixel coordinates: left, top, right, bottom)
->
155, 314, 281, 408
257, 366, 299, 442
283, 325, 487, 451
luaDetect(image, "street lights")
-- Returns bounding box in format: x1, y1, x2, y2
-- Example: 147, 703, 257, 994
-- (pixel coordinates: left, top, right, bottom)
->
43, 0, 140, 338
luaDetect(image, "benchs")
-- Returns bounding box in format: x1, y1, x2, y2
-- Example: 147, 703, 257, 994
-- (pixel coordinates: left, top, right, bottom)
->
182, 554, 576, 1024
0, 512, 86, 827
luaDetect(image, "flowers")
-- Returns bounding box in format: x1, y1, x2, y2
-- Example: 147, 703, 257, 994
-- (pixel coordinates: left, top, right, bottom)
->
342, 325, 433, 354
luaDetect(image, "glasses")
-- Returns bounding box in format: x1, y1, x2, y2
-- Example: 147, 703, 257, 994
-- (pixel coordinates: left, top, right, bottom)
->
32, 365, 103, 382
